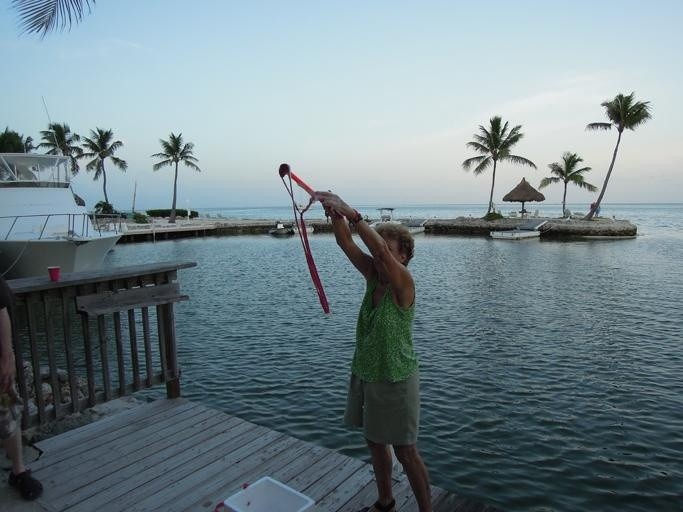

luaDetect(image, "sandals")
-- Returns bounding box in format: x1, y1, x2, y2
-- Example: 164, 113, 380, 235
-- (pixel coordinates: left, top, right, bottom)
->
7, 469, 43, 500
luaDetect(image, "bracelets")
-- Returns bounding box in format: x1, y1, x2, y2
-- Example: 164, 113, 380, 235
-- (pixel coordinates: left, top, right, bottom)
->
348, 209, 363, 226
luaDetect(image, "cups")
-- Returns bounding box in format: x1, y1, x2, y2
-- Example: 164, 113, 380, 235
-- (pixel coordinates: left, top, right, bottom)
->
47, 266, 61, 282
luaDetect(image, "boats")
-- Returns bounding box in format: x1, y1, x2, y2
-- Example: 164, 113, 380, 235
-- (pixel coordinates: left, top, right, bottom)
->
0, 96, 123, 282
368, 207, 401, 229
268, 221, 295, 235
309, 216, 332, 232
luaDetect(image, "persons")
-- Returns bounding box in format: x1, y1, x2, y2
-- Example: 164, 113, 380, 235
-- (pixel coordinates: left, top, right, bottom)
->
308, 189, 433, 511
0, 275, 45, 502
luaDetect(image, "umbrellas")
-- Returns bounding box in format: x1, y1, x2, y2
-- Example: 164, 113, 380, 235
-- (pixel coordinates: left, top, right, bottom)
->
504, 177, 547, 215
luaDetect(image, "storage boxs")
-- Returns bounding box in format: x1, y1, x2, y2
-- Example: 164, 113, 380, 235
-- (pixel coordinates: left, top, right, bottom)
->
214, 475, 317, 512
